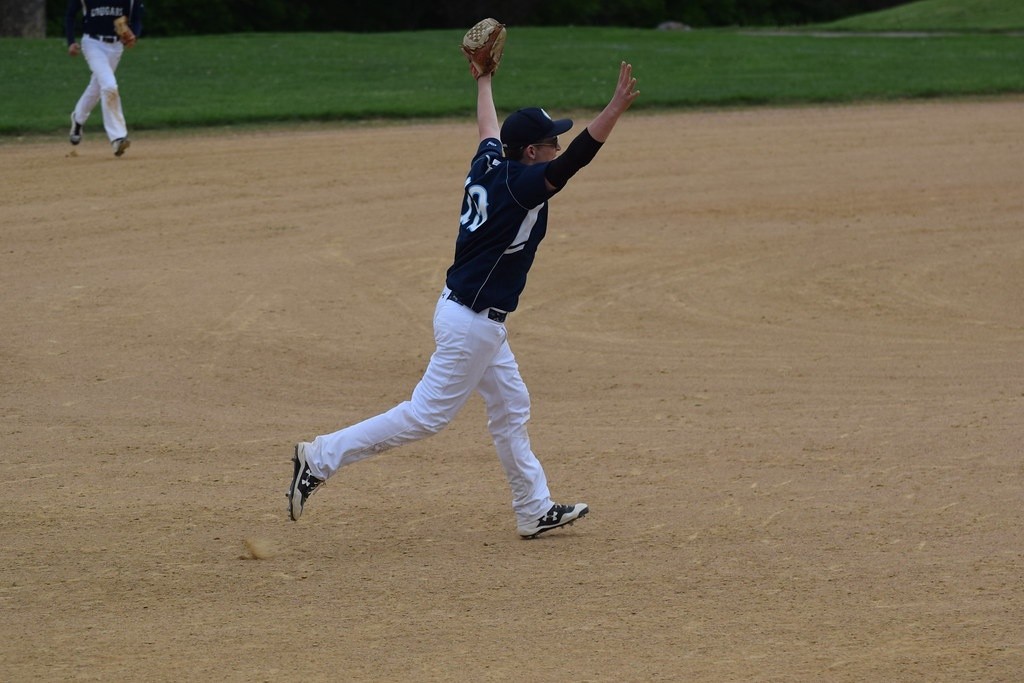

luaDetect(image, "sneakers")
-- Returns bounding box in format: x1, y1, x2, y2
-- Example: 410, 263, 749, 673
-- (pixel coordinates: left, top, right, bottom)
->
69, 111, 83, 145
285, 442, 325, 521
111, 137, 131, 156
518, 503, 589, 540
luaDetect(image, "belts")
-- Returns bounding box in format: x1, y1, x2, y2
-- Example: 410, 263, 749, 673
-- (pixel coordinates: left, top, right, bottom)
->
89, 34, 119, 43
447, 291, 507, 323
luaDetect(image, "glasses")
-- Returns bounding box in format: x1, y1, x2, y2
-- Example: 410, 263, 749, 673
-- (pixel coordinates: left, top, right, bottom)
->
520, 136, 558, 150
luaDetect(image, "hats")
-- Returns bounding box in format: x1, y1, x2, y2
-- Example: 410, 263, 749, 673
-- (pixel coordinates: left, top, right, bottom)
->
500, 107, 573, 156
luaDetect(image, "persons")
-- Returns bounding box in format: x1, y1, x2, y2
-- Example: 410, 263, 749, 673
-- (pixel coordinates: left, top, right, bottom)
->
285, 18, 639, 539
64, 0, 145, 157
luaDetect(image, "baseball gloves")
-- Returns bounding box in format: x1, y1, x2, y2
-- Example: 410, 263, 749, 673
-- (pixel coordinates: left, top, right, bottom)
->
459, 18, 507, 80
113, 15, 135, 49
